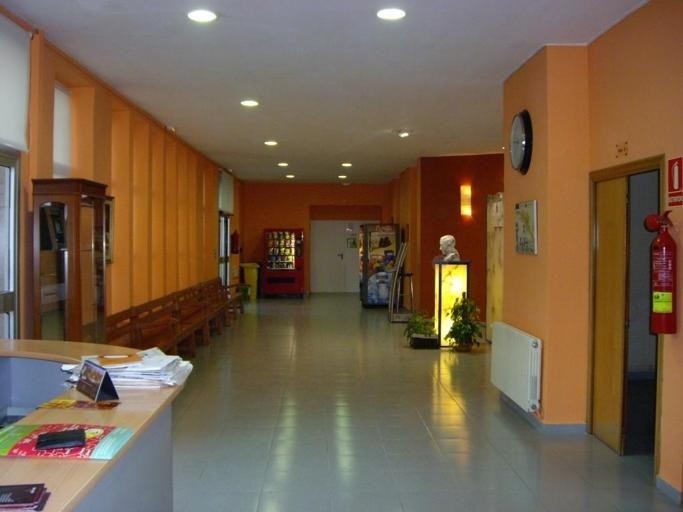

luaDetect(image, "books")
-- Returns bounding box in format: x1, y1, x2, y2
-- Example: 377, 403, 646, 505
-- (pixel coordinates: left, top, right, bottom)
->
61, 344, 193, 390
0, 422, 134, 460
0, 483, 52, 512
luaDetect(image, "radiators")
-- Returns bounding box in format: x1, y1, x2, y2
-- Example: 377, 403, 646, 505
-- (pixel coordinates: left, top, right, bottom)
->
490, 321, 542, 414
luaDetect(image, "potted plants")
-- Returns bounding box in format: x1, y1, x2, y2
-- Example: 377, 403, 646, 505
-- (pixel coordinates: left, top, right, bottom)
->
445, 292, 488, 352
403, 310, 439, 349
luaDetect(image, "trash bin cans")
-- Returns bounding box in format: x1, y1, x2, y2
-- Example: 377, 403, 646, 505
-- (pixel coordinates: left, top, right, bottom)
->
240, 262, 260, 300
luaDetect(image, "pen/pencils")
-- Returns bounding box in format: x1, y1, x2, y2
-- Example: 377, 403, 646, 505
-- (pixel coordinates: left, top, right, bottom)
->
99, 355, 131, 358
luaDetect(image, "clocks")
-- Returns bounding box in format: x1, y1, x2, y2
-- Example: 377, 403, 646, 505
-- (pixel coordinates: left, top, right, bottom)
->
509, 108, 533, 176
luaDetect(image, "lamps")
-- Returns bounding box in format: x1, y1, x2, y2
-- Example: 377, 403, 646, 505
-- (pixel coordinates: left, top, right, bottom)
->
460, 183, 472, 217
434, 262, 469, 347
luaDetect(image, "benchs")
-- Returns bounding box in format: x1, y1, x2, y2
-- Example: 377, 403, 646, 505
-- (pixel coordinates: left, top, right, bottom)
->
107, 274, 251, 361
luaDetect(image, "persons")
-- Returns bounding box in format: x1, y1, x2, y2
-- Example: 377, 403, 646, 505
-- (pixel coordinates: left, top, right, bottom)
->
439, 235, 461, 262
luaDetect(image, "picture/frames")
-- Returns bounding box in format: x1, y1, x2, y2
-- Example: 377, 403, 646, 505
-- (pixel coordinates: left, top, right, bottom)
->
104, 195, 115, 265
514, 199, 537, 255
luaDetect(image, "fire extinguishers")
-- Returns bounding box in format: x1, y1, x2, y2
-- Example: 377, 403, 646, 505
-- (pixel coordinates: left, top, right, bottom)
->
644, 210, 678, 334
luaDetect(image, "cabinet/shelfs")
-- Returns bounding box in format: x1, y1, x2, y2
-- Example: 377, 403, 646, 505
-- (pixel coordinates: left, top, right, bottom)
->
30, 176, 108, 343
